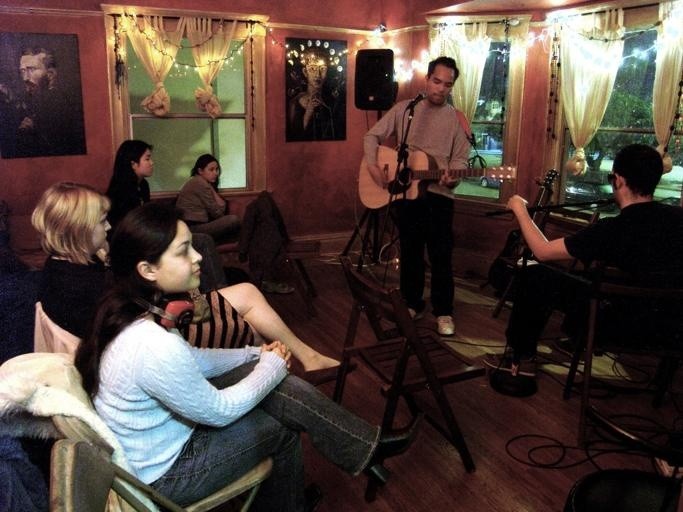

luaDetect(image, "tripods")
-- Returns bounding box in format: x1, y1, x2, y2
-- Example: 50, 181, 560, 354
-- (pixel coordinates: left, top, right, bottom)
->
341, 112, 430, 273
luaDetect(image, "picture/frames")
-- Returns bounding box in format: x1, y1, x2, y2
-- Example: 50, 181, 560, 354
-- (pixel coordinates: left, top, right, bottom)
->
0, 31, 87, 159
285, 37, 347, 142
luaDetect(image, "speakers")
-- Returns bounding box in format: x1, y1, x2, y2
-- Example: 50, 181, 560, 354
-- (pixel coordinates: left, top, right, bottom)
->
355, 49, 394, 111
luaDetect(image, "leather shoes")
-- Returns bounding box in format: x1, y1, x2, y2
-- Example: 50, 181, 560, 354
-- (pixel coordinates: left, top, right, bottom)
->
302, 361, 357, 387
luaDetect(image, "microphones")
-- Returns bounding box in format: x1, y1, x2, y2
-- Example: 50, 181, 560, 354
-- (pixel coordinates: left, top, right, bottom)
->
408, 90, 428, 109
456, 112, 472, 138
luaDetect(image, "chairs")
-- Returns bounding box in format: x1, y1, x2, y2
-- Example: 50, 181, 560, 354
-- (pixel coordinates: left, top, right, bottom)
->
248, 190, 319, 318
35, 301, 83, 354
563, 262, 683, 451
48, 414, 274, 512
332, 254, 486, 504
192, 232, 228, 294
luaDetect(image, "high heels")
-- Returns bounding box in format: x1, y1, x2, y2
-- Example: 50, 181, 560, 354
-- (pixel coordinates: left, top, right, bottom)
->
362, 412, 424, 486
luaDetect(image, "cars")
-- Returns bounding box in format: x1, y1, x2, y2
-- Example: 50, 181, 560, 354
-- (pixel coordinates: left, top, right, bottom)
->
563, 170, 617, 213
474, 100, 499, 118
460, 149, 502, 189
657, 197, 680, 207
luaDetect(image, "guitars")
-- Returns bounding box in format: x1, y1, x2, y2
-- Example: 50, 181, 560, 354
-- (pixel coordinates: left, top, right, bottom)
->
359, 144, 517, 211
488, 169, 558, 300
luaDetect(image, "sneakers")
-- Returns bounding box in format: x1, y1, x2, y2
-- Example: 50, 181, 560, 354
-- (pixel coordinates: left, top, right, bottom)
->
552, 337, 592, 365
261, 281, 295, 293
482, 353, 539, 377
437, 315, 455, 335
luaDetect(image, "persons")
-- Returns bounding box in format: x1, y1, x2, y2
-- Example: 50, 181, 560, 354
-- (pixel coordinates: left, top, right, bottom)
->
476, 142, 683, 378
289, 47, 337, 133
173, 151, 241, 236
363, 55, 474, 339
15, 45, 84, 156
29, 179, 358, 385
73, 201, 429, 508
105, 138, 156, 228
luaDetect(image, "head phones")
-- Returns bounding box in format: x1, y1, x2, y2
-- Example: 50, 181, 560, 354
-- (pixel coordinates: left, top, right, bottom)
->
134, 292, 195, 329
